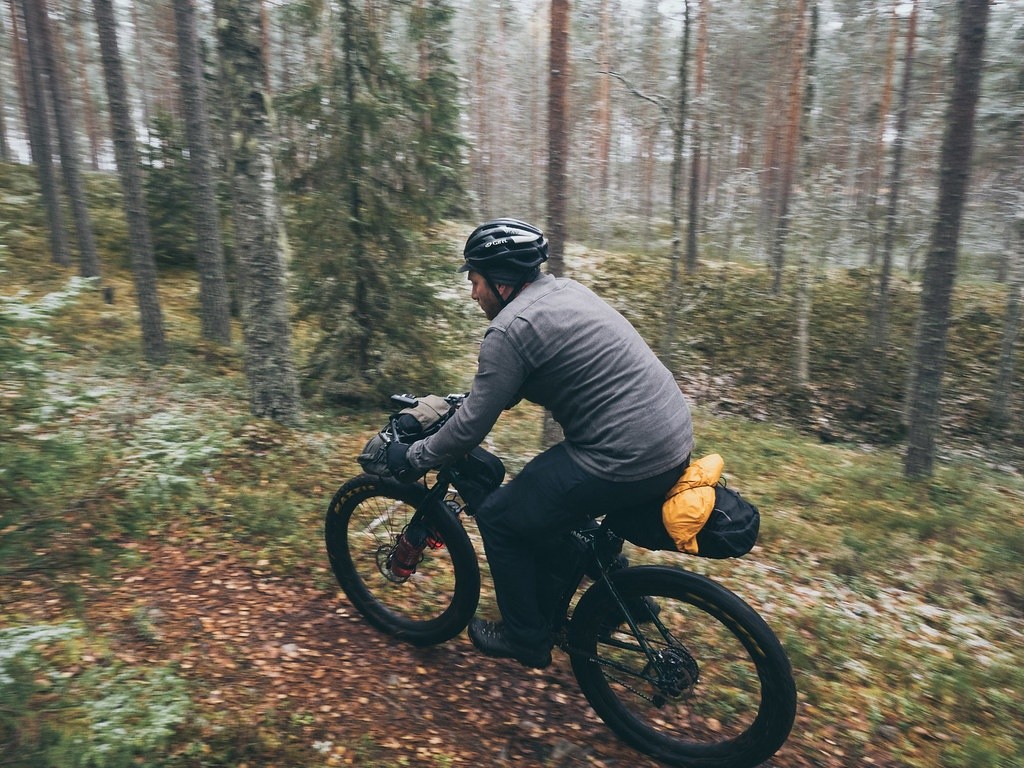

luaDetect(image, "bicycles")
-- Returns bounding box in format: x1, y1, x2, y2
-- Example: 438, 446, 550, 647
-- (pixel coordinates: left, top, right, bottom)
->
321, 391, 797, 768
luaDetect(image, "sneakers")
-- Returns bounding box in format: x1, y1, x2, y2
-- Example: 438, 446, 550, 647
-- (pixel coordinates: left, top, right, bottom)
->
621, 594, 661, 624
468, 617, 552, 670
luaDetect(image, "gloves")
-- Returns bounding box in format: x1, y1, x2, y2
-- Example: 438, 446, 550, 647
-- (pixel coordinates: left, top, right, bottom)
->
386, 443, 422, 486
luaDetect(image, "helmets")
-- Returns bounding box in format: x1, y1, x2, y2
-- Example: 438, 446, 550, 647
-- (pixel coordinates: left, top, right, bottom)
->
456, 218, 549, 285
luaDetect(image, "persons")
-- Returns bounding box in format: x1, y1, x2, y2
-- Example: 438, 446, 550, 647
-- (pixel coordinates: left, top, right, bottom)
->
386, 217, 697, 669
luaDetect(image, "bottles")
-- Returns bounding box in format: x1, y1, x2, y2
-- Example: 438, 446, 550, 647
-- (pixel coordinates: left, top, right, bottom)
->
426, 499, 463, 550
392, 527, 426, 578
